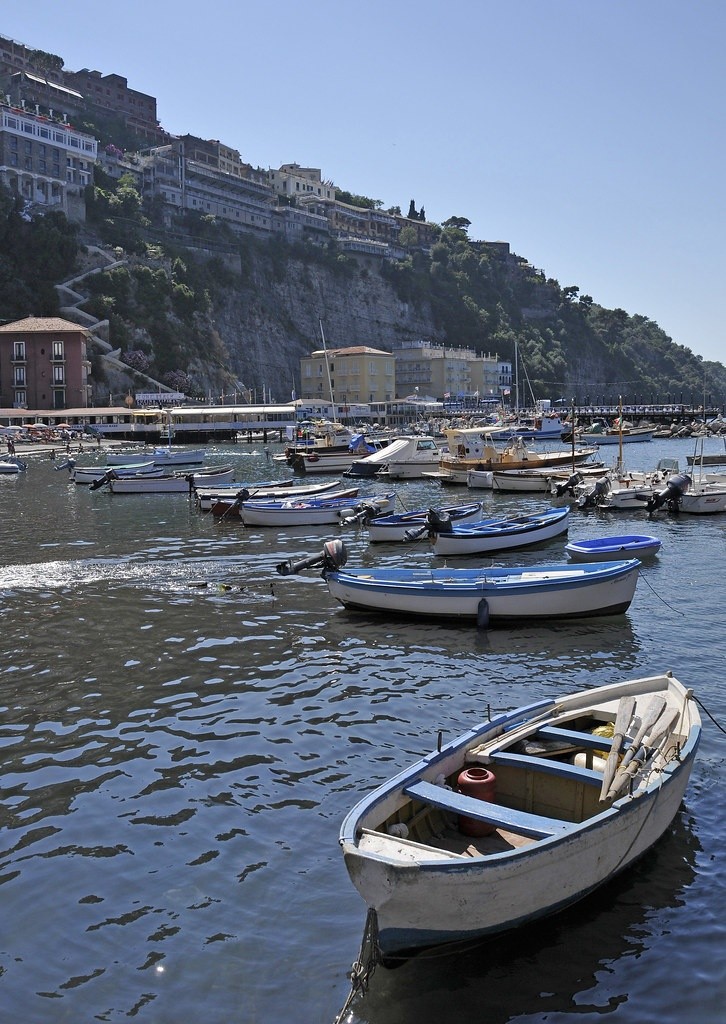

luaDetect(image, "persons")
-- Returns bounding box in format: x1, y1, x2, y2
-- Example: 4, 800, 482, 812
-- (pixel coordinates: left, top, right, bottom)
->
66, 446, 70, 453
97, 436, 100, 445
7, 440, 15, 456
49, 449, 55, 460
79, 443, 83, 453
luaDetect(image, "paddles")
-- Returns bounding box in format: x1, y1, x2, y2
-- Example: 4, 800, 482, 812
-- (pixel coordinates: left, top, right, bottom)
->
606, 694, 667, 803
612, 706, 680, 794
598, 694, 636, 805
401, 503, 477, 521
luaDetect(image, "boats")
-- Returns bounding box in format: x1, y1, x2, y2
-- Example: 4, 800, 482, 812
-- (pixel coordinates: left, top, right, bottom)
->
562, 534, 663, 564
338, 503, 483, 545
403, 505, 571, 558
465, 340, 726, 520
0, 453, 20, 474
104, 408, 209, 466
88, 463, 237, 495
418, 422, 602, 486
52, 457, 156, 480
72, 467, 165, 485
289, 437, 400, 475
184, 472, 398, 529
338, 668, 704, 954
275, 540, 646, 623
340, 434, 451, 479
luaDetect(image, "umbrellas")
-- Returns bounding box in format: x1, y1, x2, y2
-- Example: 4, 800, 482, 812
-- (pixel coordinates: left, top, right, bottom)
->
0, 423, 47, 433
57, 423, 71, 431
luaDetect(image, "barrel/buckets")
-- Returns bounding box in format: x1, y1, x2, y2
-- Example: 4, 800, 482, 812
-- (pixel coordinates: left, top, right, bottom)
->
458, 768, 497, 837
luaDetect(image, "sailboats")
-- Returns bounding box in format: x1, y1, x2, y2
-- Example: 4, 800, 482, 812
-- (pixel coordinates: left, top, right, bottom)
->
281, 316, 396, 456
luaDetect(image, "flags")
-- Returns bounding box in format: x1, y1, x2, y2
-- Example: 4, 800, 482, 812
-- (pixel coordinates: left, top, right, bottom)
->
504, 389, 510, 395
